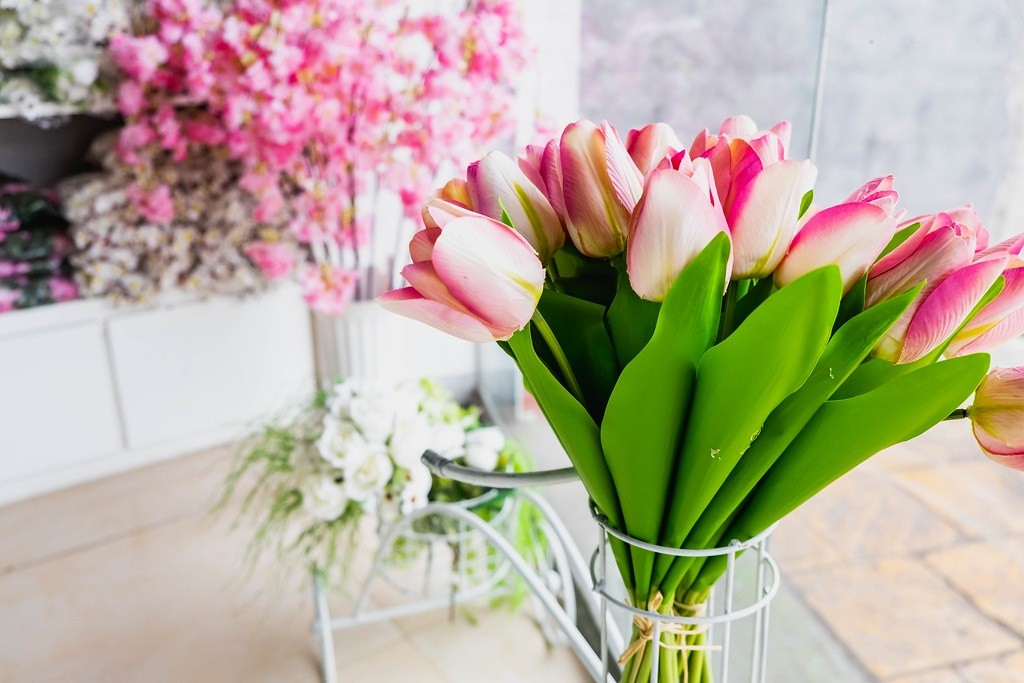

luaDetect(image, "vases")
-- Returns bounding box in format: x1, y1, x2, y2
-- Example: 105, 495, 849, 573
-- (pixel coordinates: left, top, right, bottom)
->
581, 499, 778, 683
311, 298, 388, 392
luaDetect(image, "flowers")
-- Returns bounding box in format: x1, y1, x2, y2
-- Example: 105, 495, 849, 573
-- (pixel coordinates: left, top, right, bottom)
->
110, 0, 528, 310
214, 381, 546, 608
380, 115, 1024, 547
